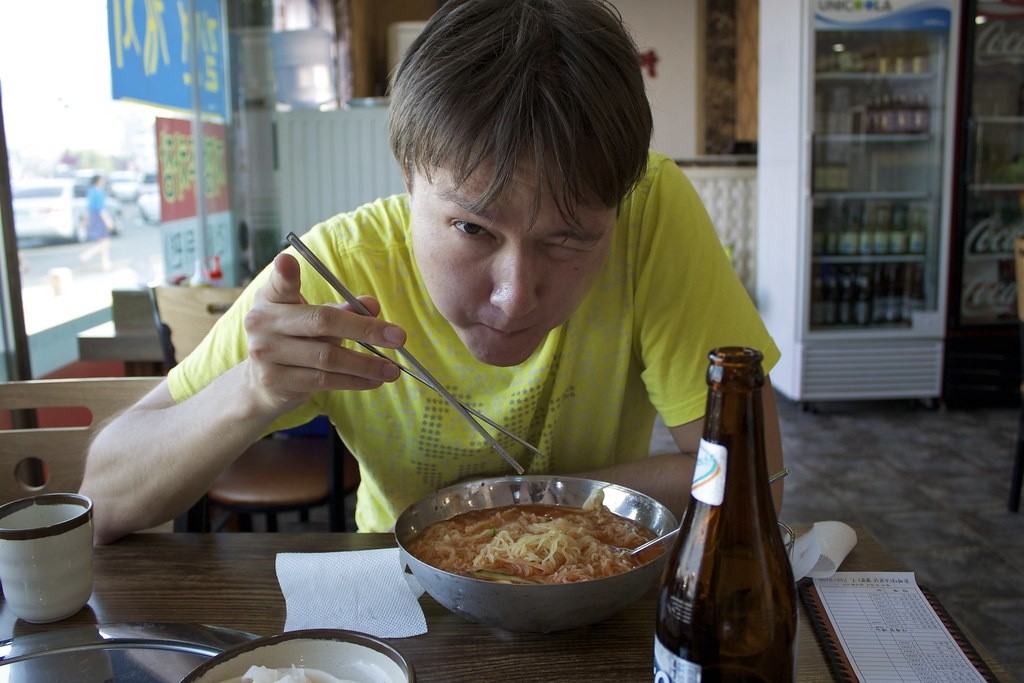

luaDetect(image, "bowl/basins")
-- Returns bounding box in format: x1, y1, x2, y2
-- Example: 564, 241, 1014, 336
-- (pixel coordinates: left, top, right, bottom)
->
395, 475, 679, 634
177, 628, 417, 683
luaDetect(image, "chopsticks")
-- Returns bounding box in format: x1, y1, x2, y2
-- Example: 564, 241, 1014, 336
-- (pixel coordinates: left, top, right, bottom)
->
287, 232, 544, 474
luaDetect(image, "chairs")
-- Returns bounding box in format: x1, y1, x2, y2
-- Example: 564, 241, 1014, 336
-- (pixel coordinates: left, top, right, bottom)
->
145, 285, 361, 533
0, 375, 188, 533
1008, 236, 1024, 513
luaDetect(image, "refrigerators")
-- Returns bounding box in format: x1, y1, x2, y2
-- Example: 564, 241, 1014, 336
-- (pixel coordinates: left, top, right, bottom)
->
755, 0, 1024, 410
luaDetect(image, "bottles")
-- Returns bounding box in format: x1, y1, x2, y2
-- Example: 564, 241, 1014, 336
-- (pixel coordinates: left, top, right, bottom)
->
815, 199, 927, 256
653, 347, 797, 683
189, 256, 223, 288
812, 264, 926, 326
819, 32, 929, 132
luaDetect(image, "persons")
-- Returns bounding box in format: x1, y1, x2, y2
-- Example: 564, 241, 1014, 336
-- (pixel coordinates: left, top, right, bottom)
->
80, 173, 114, 272
77, 0, 786, 546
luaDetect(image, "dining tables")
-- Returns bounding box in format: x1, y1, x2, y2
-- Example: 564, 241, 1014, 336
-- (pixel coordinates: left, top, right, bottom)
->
77, 313, 164, 365
0, 519, 1014, 682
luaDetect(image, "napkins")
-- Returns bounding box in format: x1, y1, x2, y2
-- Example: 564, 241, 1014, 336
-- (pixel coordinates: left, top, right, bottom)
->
272, 548, 429, 639
784, 521, 857, 583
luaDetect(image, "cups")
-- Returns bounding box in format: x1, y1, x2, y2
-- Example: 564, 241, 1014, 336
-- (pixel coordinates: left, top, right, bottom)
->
0, 493, 95, 624
